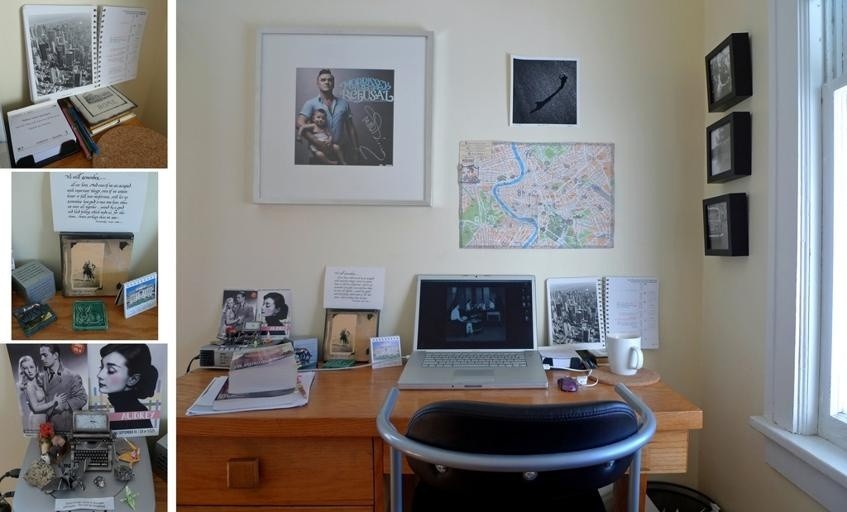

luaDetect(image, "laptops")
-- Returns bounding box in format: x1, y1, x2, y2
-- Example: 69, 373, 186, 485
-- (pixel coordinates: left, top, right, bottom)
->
397, 274, 549, 390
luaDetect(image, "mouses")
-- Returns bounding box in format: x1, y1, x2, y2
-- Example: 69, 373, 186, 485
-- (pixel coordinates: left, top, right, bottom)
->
556, 376, 578, 392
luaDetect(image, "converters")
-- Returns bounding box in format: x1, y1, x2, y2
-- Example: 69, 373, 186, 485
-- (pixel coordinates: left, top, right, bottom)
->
575, 376, 588, 385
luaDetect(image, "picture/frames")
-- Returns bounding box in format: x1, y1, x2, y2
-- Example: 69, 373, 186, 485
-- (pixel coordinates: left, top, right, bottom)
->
704, 32, 753, 112
252, 28, 432, 207
320, 308, 380, 363
706, 112, 751, 184
702, 193, 749, 257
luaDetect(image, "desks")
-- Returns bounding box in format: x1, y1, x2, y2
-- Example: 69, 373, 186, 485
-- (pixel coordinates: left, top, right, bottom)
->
178, 357, 703, 512
13, 435, 157, 511
42, 117, 144, 168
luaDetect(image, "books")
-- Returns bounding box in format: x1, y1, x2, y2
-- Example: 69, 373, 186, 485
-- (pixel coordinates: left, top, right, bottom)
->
68, 86, 138, 138
546, 273, 659, 351
226, 339, 296, 394
22, 4, 148, 103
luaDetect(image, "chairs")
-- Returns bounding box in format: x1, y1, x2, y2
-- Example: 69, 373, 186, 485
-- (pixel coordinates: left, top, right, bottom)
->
377, 382, 657, 512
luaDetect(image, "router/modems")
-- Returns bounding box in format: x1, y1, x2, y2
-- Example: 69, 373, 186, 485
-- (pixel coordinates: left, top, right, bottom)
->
199, 344, 233, 370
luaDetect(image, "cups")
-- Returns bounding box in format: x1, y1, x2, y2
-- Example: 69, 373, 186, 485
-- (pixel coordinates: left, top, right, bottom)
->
606, 332, 644, 376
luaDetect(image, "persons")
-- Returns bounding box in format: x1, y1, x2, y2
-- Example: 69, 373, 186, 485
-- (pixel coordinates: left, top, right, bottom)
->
216, 297, 239, 340
299, 69, 349, 164
17, 356, 71, 432
97, 344, 158, 429
450, 297, 496, 335
296, 109, 347, 165
233, 292, 254, 325
261, 293, 288, 335
37, 345, 87, 433
